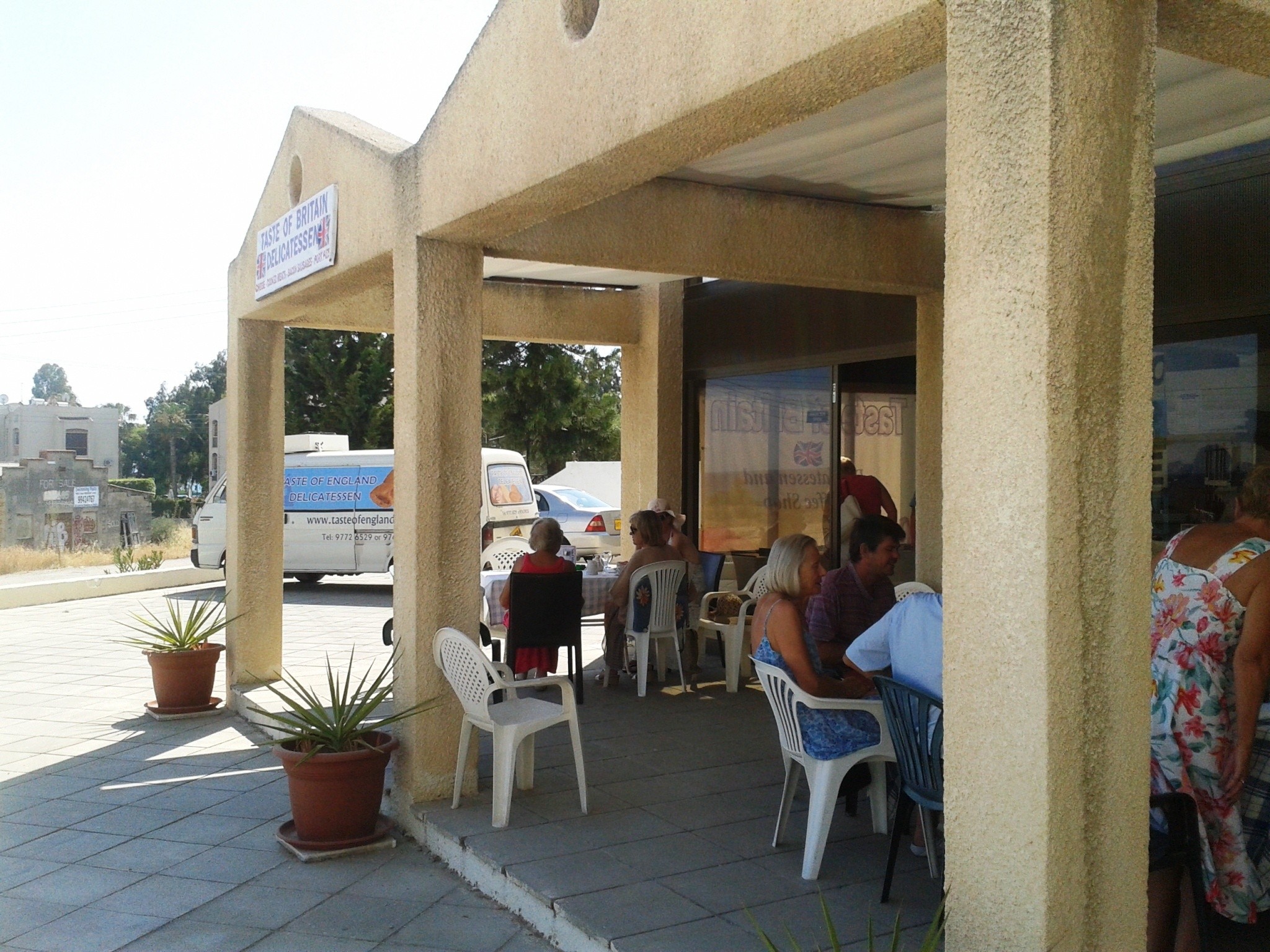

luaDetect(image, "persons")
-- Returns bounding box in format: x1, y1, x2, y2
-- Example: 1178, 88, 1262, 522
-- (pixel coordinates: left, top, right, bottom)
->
750, 455, 945, 858
499, 518, 576, 691
594, 498, 708, 686
1150, 462, 1270, 952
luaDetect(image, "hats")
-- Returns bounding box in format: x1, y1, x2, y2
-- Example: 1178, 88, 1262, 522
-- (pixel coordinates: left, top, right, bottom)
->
651, 498, 686, 529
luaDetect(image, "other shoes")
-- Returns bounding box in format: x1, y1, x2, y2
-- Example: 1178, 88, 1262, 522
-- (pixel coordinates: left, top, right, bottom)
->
533, 672, 547, 691
516, 672, 527, 681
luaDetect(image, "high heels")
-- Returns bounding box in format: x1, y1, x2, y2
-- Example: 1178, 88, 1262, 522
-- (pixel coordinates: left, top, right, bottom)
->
594, 669, 620, 685
647, 670, 655, 683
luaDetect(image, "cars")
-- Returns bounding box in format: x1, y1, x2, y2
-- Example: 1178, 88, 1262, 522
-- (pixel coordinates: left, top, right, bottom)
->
529, 484, 622, 557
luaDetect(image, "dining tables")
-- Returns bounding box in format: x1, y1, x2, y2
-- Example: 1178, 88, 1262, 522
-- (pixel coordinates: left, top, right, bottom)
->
479, 570, 634, 702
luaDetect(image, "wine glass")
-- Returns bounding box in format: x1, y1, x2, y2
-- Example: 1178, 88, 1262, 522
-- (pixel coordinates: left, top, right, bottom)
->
601, 550, 612, 568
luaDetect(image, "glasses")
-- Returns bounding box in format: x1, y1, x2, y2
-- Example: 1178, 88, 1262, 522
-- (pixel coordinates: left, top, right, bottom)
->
660, 514, 668, 520
630, 526, 638, 535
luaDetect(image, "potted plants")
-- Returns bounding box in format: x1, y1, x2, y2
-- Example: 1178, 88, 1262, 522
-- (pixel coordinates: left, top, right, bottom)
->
243, 636, 446, 849
109, 587, 250, 714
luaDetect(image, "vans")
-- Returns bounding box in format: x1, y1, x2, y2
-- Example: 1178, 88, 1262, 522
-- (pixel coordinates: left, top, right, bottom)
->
190, 432, 541, 583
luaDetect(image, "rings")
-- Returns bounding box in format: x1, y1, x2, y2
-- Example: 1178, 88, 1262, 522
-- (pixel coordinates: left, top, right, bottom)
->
1239, 778, 1245, 785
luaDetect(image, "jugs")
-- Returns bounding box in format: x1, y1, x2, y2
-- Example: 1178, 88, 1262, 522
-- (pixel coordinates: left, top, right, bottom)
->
586, 560, 598, 575
592, 558, 604, 572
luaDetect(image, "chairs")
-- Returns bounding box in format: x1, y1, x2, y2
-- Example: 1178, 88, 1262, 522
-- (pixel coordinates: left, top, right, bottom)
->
432, 550, 1209, 952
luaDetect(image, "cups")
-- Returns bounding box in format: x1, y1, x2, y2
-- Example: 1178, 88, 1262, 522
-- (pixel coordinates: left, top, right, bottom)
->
617, 564, 626, 576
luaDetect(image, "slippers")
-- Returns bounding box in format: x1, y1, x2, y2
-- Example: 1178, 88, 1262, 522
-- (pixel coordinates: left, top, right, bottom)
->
621, 664, 636, 674
910, 829, 945, 856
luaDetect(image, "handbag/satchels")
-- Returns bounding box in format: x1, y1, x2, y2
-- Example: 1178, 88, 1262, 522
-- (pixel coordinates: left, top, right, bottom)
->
840, 481, 862, 545
